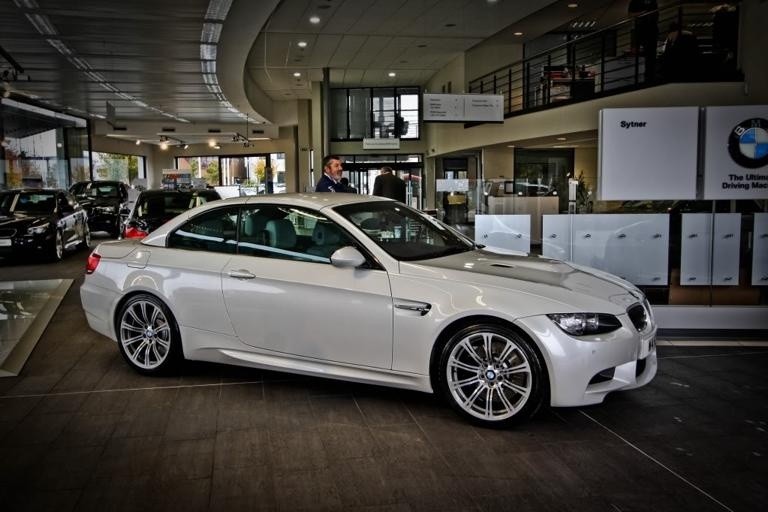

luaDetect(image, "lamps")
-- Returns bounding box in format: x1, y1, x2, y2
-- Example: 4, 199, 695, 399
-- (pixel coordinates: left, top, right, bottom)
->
159, 135, 189, 151
233, 133, 251, 150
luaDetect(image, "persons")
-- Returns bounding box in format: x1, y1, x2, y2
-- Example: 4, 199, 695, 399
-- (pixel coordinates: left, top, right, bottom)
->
339, 178, 348, 186
621, 1, 660, 82
371, 165, 406, 205
314, 153, 358, 195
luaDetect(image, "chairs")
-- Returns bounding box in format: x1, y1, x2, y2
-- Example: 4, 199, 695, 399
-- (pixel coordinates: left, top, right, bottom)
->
245, 212, 274, 252
262, 218, 306, 254
306, 222, 344, 258
193, 219, 226, 253
